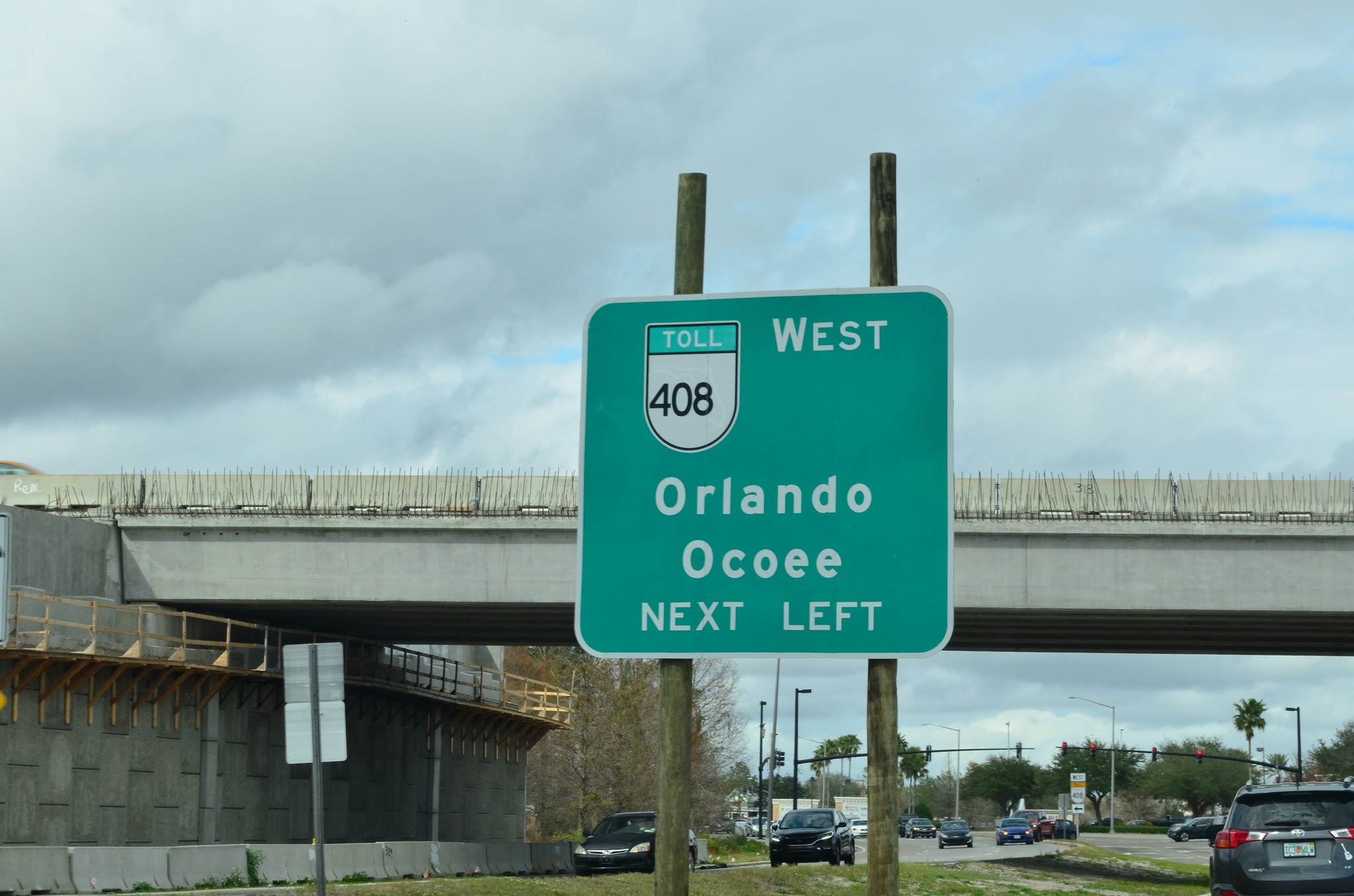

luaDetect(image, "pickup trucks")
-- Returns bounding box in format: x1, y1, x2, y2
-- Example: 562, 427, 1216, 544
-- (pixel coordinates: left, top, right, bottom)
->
1009, 809, 1057, 842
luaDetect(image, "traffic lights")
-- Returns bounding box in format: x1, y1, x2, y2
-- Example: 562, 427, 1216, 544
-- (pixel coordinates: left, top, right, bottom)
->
1090, 743, 1097, 759
1062, 742, 1068, 756
1152, 747, 1157, 762
774, 748, 785, 767
1196, 750, 1204, 765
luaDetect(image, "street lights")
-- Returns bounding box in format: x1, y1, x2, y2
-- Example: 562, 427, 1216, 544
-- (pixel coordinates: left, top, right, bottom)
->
1068, 696, 1116, 833
1285, 707, 1303, 782
757, 700, 766, 840
1006, 721, 1010, 759
790, 687, 811, 866
1120, 729, 1124, 757
921, 723, 960, 821
1256, 748, 1265, 785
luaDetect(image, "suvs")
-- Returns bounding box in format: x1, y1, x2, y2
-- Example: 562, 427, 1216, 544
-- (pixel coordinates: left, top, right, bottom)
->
1209, 776, 1354, 896
1086, 817, 1125, 826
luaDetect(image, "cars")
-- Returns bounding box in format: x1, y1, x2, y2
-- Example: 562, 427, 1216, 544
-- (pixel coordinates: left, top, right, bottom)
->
769, 807, 856, 868
898, 814, 936, 839
573, 811, 699, 877
734, 818, 747, 827
1055, 819, 1080, 840
703, 815, 735, 834
0, 460, 88, 512
846, 816, 868, 838
746, 817, 783, 837
995, 818, 1036, 846
1126, 815, 1228, 846
936, 820, 973, 849
